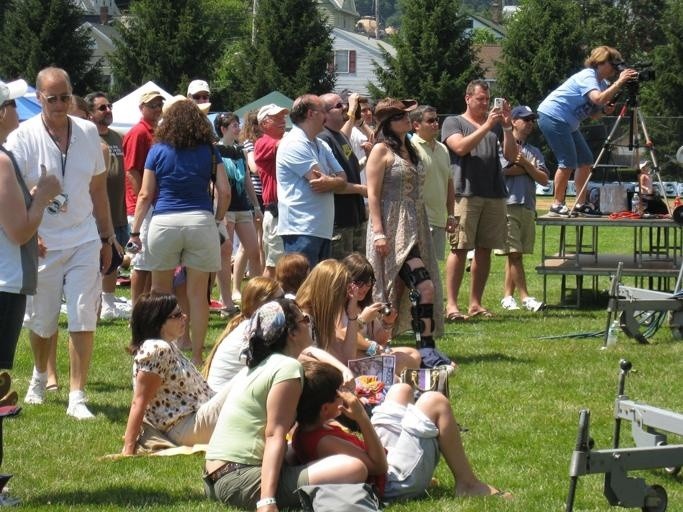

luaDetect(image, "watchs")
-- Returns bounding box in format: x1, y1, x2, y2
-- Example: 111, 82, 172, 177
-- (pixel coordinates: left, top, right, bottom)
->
101, 236, 114, 246
129, 232, 141, 237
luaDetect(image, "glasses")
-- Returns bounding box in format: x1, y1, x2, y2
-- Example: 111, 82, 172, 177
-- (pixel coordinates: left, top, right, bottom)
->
192, 94, 210, 102
146, 101, 167, 107
325, 101, 343, 111
90, 101, 114, 112
0, 98, 18, 108
37, 88, 73, 105
165, 310, 184, 320
522, 116, 537, 123
352, 278, 378, 288
298, 314, 312, 326
419, 116, 442, 125
219, 110, 236, 126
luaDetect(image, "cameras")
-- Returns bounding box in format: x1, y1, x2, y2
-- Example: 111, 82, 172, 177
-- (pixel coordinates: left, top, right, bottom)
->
47, 194, 69, 216
607, 62, 656, 109
378, 303, 392, 315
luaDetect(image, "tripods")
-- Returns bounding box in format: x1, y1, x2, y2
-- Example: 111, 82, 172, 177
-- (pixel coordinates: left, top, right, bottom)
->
568, 101, 673, 218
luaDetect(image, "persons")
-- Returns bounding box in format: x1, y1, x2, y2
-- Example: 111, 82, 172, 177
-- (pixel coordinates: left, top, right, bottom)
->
186, 80, 210, 119
2, 67, 113, 421
124, 92, 166, 309
408, 105, 455, 261
334, 255, 399, 348
96, 292, 232, 463
127, 101, 231, 369
348, 91, 374, 171
83, 92, 126, 320
202, 276, 285, 394
536, 46, 638, 218
241, 109, 265, 280
640, 164, 656, 215
210, 112, 263, 318
292, 360, 501, 499
205, 298, 368, 510
276, 95, 346, 276
339, 94, 376, 258
442, 80, 517, 321
0, 79, 62, 417
497, 106, 549, 312
43, 94, 110, 390
273, 254, 310, 303
254, 105, 286, 282
366, 101, 454, 369
315, 93, 368, 263
295, 258, 360, 369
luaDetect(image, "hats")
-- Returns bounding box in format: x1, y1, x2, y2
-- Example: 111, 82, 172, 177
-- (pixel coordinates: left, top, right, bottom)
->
372, 97, 420, 118
137, 90, 167, 108
509, 105, 540, 123
186, 78, 211, 96
0, 78, 29, 105
339, 88, 360, 103
638, 158, 652, 170
256, 102, 290, 126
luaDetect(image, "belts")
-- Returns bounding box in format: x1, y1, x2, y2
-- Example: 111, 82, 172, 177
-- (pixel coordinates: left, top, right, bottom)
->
201, 460, 252, 486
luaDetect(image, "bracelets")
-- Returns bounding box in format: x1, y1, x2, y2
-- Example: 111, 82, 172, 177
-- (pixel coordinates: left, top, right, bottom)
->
447, 215, 454, 219
502, 126, 512, 131
373, 234, 387, 241
214, 218, 223, 224
254, 206, 260, 210
129, 231, 141, 237
356, 119, 366, 130
255, 497, 276, 507
347, 314, 358, 321
447, 215, 456, 220
255, 497, 277, 509
382, 319, 393, 333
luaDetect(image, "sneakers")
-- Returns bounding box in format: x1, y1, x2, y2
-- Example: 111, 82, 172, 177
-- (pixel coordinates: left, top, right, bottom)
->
207, 299, 227, 313
65, 398, 96, 420
522, 298, 544, 314
230, 290, 244, 306
547, 202, 579, 220
571, 200, 604, 219
500, 295, 520, 310
23, 375, 49, 405
100, 304, 116, 322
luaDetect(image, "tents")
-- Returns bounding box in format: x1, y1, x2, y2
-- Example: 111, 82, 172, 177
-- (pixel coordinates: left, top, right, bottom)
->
231, 91, 296, 130
108, 81, 177, 135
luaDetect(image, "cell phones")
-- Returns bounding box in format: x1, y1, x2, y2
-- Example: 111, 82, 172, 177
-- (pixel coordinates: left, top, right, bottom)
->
494, 97, 504, 109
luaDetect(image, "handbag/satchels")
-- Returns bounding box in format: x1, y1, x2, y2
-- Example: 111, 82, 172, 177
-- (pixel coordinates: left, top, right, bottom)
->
400, 367, 451, 404
599, 153, 628, 217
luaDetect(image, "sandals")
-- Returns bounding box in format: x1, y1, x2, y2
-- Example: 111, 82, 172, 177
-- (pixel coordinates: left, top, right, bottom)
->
467, 307, 498, 318
445, 310, 471, 324
492, 485, 514, 501
